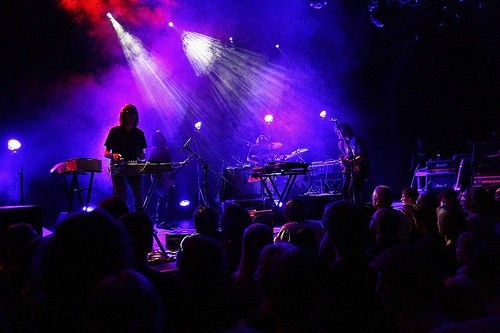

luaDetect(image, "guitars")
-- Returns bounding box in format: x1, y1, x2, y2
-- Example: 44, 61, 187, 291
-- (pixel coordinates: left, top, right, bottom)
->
151, 152, 196, 196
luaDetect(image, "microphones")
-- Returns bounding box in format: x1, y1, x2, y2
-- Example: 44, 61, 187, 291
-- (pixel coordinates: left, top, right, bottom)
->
181, 137, 193, 152
329, 116, 338, 121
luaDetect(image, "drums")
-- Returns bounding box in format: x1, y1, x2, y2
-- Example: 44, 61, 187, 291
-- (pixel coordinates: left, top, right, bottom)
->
223, 167, 262, 199
288, 168, 311, 194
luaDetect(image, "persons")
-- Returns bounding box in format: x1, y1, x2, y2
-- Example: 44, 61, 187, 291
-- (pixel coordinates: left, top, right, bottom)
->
149, 132, 180, 228
104, 104, 148, 210
0, 170, 500, 332
336, 122, 367, 201
247, 135, 268, 167
407, 138, 433, 177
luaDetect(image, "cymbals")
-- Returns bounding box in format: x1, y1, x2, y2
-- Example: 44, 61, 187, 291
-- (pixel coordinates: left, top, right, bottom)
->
287, 148, 308, 159
265, 142, 283, 150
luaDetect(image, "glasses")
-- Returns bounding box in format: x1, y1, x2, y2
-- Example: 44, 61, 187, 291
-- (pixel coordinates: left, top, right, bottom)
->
124, 110, 137, 115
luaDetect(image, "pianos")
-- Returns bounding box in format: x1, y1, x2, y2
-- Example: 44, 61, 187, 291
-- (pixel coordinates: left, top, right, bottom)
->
110, 160, 175, 176
50, 158, 102, 174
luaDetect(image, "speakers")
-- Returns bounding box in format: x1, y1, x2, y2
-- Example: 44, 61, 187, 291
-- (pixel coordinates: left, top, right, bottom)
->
0, 205, 42, 235
